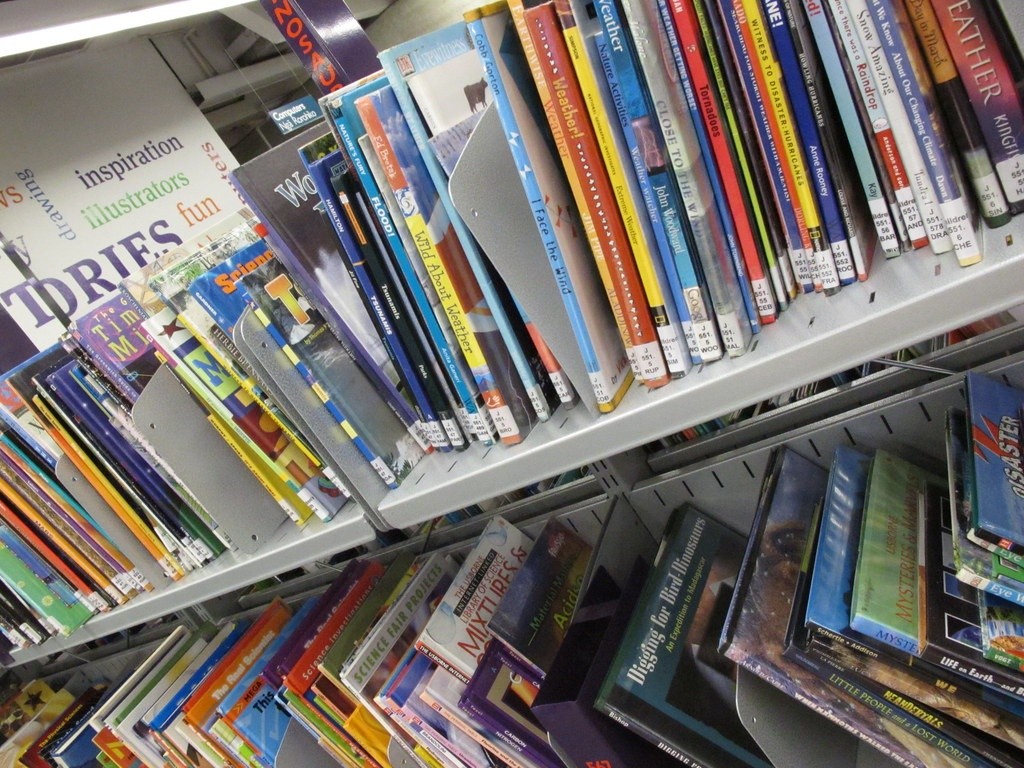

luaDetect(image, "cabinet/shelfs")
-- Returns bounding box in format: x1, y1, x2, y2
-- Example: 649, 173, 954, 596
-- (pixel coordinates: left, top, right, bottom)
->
0, 2, 1024, 768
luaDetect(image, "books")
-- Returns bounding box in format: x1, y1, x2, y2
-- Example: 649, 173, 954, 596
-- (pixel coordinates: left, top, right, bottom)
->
2, 0, 1024, 764
